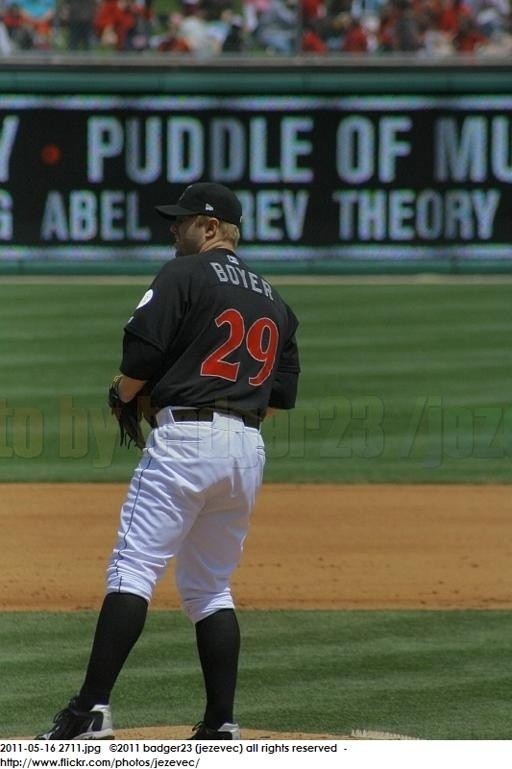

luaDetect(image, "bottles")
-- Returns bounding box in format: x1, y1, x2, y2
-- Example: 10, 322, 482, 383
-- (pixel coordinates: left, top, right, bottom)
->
154, 183, 242, 225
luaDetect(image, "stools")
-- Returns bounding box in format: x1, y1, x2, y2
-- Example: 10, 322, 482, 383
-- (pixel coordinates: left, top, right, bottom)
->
152, 408, 263, 431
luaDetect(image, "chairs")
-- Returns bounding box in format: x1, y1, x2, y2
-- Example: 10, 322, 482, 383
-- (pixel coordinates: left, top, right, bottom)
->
32, 695, 115, 740
185, 721, 240, 740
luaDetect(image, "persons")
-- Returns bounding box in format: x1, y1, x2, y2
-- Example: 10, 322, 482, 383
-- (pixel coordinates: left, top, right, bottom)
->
29, 181, 300, 741
